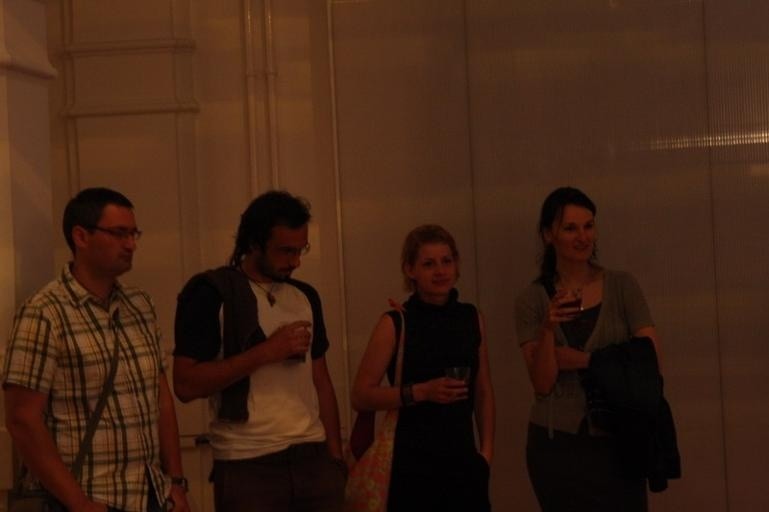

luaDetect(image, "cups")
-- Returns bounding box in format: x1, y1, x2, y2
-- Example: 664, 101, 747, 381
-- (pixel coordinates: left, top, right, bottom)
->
556, 286, 584, 316
443, 366, 470, 396
288, 325, 307, 362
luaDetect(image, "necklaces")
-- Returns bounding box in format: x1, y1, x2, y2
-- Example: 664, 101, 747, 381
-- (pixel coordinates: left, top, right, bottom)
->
239, 263, 277, 307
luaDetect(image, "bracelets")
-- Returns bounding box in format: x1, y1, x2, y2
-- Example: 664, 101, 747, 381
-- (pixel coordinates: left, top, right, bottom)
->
172, 478, 188, 488
400, 383, 417, 407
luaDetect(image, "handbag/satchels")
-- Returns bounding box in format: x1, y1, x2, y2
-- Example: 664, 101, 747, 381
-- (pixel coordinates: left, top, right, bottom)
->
342, 411, 399, 511
7, 488, 64, 510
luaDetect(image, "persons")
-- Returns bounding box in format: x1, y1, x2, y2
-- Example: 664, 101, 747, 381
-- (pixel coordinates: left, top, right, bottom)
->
2, 187, 192, 512
172, 191, 348, 512
349, 222, 506, 511
514, 187, 683, 512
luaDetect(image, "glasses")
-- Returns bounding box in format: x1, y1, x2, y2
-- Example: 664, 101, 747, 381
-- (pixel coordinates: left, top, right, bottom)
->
93, 226, 142, 240
